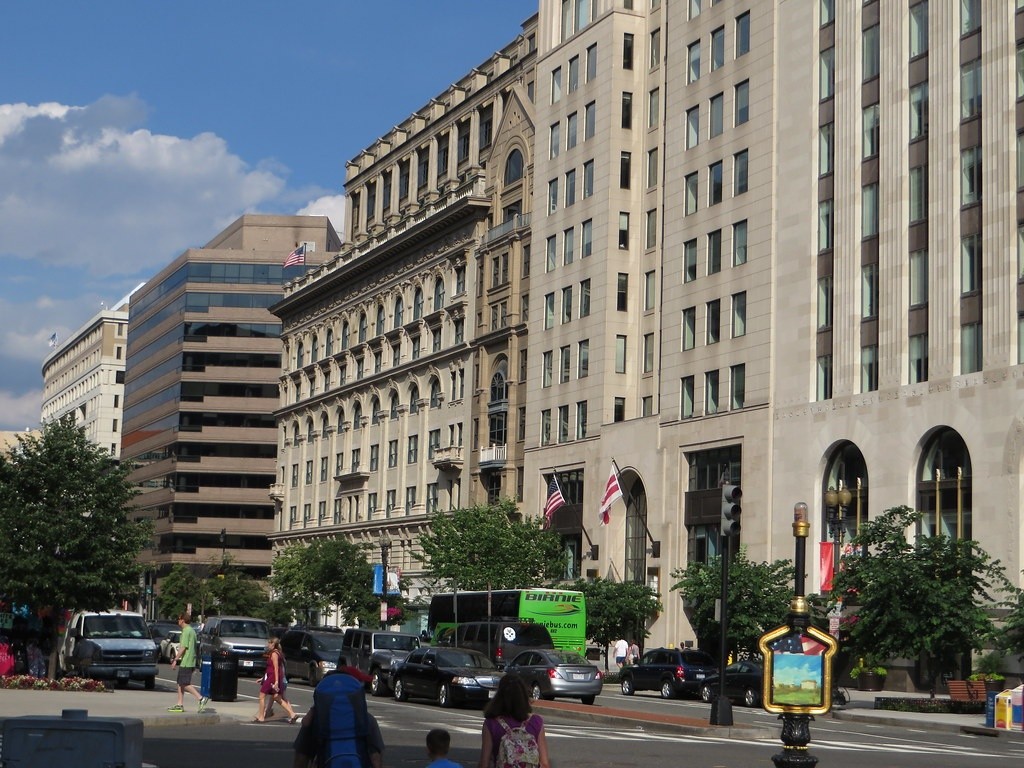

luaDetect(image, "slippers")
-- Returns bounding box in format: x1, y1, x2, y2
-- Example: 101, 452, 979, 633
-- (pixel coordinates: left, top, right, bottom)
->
288, 714, 300, 724
250, 717, 265, 723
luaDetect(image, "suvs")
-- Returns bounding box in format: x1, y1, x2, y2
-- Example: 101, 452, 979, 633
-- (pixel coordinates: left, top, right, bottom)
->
58, 611, 158, 688
336, 629, 422, 696
617, 648, 718, 699
438, 616, 555, 670
198, 615, 274, 677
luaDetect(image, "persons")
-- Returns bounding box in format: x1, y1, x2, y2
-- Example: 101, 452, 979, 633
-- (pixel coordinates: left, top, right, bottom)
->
293, 665, 387, 768
612, 638, 641, 670
478, 673, 551, 768
167, 612, 211, 712
252, 637, 299, 724
423, 728, 464, 768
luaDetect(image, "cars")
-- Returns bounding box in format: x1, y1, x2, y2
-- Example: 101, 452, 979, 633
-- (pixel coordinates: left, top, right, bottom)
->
392, 646, 508, 711
147, 620, 199, 663
277, 628, 345, 685
502, 648, 603, 705
703, 657, 765, 707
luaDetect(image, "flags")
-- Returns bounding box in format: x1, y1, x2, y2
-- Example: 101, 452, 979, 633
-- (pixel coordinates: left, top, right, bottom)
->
284, 246, 304, 267
599, 465, 624, 525
543, 473, 566, 530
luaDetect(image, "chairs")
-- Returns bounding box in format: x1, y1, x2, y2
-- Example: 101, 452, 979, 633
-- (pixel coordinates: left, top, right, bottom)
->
672, 655, 680, 664
222, 622, 233, 632
745, 668, 753, 673
234, 624, 243, 632
530, 654, 542, 665
726, 668, 737, 673
245, 622, 256, 632
567, 656, 578, 663
399, 639, 409, 649
653, 654, 668, 664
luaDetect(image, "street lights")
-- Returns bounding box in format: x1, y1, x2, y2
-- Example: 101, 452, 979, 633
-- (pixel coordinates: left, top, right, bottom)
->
378, 535, 392, 629
219, 527, 226, 578
827, 482, 852, 607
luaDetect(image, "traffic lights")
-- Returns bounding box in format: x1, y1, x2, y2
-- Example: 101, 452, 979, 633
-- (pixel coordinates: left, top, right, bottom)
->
719, 484, 745, 538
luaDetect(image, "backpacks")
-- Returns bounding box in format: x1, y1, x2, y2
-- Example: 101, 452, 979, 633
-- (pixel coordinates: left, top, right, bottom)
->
312, 674, 373, 768
493, 712, 540, 768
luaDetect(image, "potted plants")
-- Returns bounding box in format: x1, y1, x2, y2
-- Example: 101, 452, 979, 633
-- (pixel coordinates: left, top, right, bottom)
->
984, 671, 1007, 703
850, 666, 887, 691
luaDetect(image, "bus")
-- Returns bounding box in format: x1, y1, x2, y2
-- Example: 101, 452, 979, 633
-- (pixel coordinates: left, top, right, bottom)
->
427, 587, 590, 658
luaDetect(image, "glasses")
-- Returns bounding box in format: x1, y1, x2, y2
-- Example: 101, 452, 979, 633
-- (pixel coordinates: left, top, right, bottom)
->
268, 641, 274, 644
178, 617, 184, 620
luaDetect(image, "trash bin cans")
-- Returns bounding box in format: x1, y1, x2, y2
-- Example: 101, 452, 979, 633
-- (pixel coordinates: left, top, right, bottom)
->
210, 650, 239, 702
985, 691, 999, 728
200, 654, 211, 698
994, 690, 1012, 730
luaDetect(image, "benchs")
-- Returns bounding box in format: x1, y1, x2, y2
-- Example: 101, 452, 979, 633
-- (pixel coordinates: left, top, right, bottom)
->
947, 680, 988, 712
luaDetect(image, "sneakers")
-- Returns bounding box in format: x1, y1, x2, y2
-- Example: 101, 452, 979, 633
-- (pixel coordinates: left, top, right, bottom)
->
167, 704, 185, 712
197, 697, 209, 713
